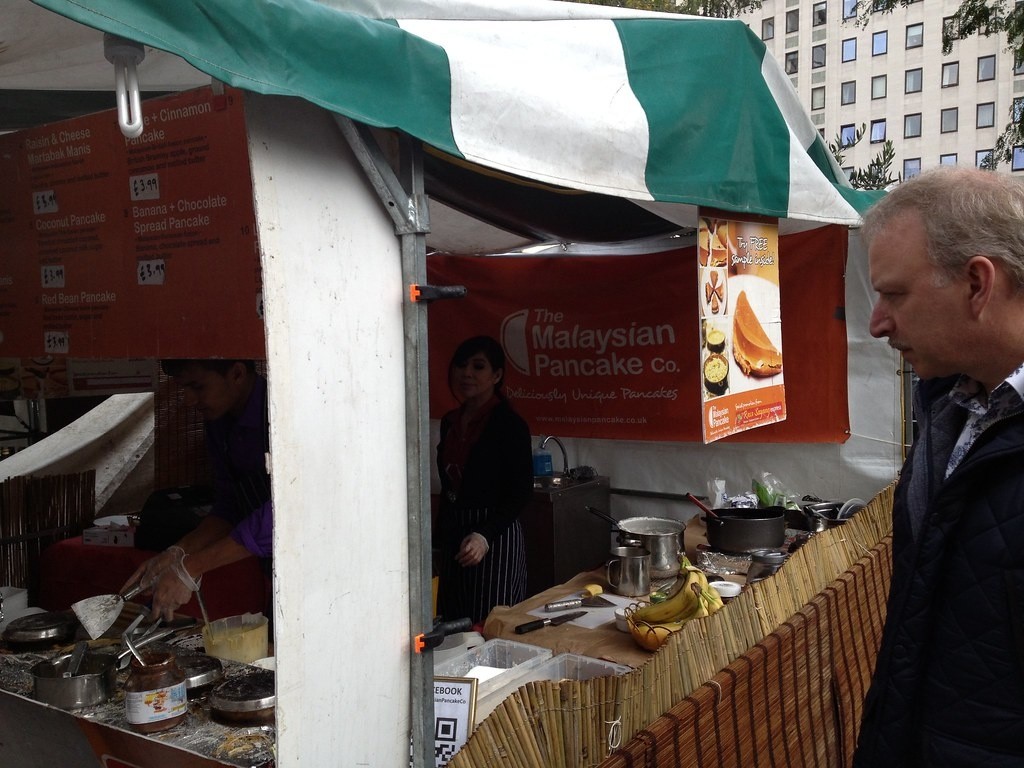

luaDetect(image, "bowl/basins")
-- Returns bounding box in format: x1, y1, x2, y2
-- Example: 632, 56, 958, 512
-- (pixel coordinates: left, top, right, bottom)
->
703, 354, 730, 392
707, 331, 726, 353
614, 609, 630, 633
254, 655, 275, 672
786, 499, 814, 527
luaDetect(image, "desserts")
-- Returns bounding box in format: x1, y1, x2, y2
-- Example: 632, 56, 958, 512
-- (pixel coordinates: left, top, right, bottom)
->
699, 221, 726, 314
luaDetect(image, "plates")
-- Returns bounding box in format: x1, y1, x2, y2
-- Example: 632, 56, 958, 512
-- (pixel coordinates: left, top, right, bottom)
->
709, 276, 784, 395
93, 515, 140, 526
837, 498, 866, 519
701, 268, 727, 315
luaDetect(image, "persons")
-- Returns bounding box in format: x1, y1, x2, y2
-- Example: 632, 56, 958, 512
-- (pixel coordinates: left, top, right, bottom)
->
432, 336, 532, 624
858, 167, 1024, 768
120, 359, 273, 623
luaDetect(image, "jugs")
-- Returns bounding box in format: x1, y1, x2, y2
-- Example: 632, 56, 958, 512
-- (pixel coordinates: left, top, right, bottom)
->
434, 632, 489, 679
203, 611, 270, 662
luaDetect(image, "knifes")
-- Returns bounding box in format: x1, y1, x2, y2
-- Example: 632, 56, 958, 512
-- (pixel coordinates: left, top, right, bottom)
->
515, 610, 587, 634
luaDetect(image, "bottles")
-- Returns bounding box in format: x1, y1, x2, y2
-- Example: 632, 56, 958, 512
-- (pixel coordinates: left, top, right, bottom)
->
123, 652, 188, 734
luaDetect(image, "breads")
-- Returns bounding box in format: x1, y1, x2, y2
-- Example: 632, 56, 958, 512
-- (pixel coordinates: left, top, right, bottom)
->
732, 291, 781, 378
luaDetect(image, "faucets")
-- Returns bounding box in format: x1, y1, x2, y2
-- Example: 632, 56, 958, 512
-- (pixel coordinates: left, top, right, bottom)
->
539, 433, 570, 476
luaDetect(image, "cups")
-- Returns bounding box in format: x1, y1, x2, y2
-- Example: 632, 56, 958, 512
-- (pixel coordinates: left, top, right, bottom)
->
606, 547, 651, 596
746, 551, 788, 583
710, 581, 742, 603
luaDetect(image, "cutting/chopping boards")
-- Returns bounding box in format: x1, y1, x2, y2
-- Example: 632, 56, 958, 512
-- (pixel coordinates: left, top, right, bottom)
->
526, 591, 644, 630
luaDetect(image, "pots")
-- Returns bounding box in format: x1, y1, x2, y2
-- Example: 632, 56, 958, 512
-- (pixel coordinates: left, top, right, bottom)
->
584, 505, 685, 578
807, 502, 845, 529
701, 506, 786, 552
31, 629, 175, 708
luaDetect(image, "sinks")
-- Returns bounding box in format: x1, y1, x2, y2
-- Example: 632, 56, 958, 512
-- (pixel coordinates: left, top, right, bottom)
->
534, 477, 543, 489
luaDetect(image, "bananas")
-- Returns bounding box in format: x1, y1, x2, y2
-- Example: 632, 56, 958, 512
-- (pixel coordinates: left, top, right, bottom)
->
633, 565, 724, 635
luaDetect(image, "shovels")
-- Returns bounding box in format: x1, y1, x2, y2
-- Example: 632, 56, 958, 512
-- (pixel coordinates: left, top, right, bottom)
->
544, 594, 618, 612
71, 572, 157, 641
